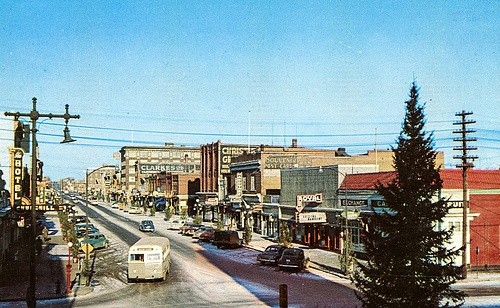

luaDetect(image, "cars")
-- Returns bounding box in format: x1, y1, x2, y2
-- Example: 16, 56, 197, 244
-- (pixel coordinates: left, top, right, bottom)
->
257, 245, 288, 265
170, 220, 220, 243
278, 249, 310, 271
71, 215, 109, 252
139, 220, 155, 232
112, 203, 142, 214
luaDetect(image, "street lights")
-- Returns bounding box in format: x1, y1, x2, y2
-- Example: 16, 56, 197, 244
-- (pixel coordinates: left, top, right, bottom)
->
85, 165, 119, 272
318, 166, 349, 276
4, 97, 81, 308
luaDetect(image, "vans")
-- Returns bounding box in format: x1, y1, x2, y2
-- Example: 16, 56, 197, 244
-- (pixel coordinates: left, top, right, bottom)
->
214, 230, 239, 249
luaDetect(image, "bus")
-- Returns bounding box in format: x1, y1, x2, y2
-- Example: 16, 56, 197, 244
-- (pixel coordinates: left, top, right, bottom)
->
128, 237, 171, 281
91, 197, 98, 205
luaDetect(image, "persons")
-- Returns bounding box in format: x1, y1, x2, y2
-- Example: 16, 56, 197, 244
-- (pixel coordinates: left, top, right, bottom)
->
36, 237, 43, 256
42, 226, 48, 241
